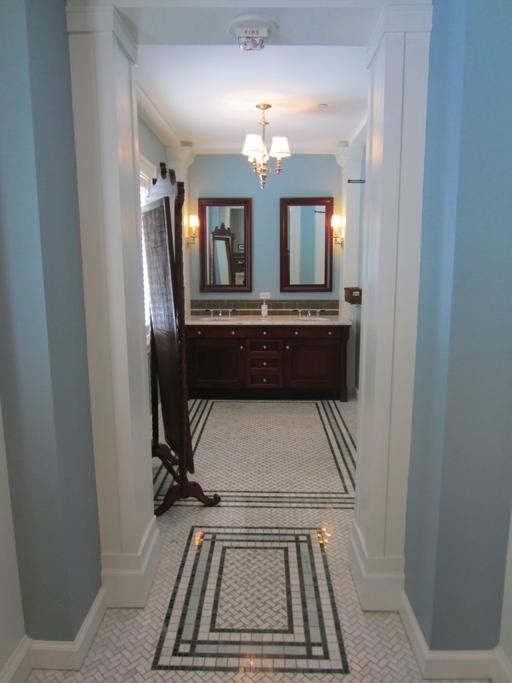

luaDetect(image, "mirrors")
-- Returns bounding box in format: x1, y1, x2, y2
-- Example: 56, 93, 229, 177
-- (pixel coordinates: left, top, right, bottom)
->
197, 197, 252, 292
143, 161, 220, 514
208, 222, 235, 287
279, 196, 334, 293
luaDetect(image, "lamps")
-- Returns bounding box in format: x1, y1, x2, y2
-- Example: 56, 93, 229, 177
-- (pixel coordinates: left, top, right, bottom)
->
330, 213, 345, 247
241, 103, 292, 190
186, 214, 201, 248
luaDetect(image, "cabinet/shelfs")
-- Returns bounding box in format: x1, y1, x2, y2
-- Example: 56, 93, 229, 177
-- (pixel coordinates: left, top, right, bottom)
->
185, 325, 350, 403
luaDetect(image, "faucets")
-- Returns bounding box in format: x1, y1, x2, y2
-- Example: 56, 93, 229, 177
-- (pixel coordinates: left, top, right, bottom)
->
217, 308, 224, 317
306, 308, 314, 317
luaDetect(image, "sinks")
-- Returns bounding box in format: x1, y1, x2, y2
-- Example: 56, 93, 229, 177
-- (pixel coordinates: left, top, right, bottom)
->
296, 316, 330, 322
203, 317, 234, 322
